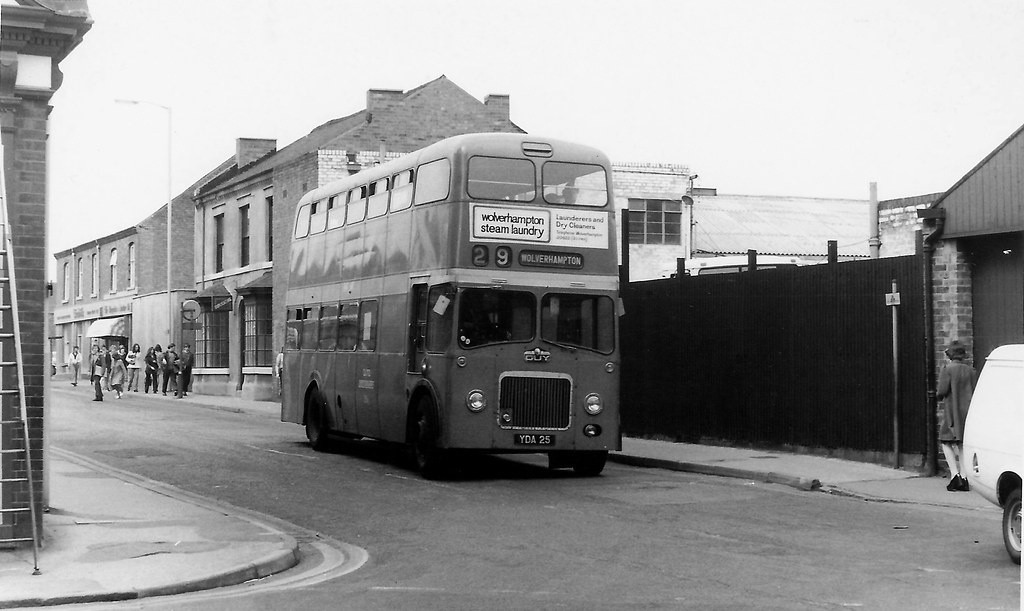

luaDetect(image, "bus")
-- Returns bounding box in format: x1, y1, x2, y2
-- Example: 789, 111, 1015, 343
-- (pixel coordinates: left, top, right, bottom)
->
283, 132, 619, 474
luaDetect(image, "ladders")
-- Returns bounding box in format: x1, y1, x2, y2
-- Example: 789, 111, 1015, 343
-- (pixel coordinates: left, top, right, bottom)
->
0, 168, 44, 575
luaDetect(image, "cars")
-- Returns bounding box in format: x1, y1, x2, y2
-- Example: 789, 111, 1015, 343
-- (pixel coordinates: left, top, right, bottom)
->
961, 344, 1024, 563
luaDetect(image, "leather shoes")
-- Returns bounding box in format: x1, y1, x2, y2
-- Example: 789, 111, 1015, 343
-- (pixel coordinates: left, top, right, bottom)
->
946, 474, 969, 491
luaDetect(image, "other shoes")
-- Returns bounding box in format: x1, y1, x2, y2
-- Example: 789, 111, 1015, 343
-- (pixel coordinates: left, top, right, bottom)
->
145, 391, 148, 393
163, 393, 167, 396
93, 398, 102, 401
116, 392, 124, 399
128, 389, 130, 391
153, 391, 158, 394
134, 390, 138, 392
174, 393, 177, 396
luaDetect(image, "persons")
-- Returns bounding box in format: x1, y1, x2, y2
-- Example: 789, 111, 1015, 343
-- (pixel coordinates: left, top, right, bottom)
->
125, 344, 142, 391
68, 346, 83, 386
92, 345, 106, 402
109, 353, 127, 398
544, 181, 579, 203
178, 343, 194, 399
154, 344, 164, 391
144, 347, 158, 393
936, 339, 976, 491
161, 343, 181, 396
90, 344, 128, 390
276, 347, 283, 397
